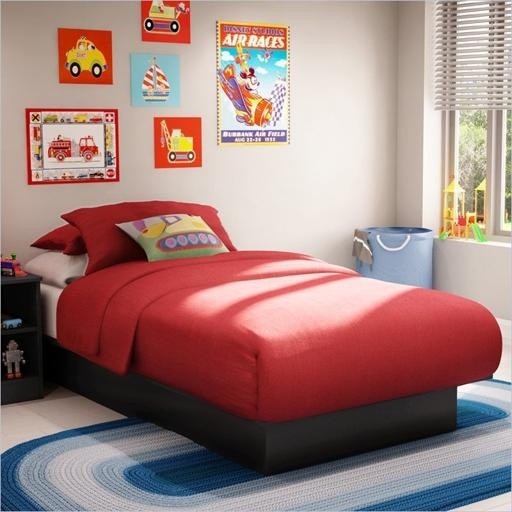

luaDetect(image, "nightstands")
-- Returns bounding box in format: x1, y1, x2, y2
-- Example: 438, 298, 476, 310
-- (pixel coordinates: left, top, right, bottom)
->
1, 271, 44, 405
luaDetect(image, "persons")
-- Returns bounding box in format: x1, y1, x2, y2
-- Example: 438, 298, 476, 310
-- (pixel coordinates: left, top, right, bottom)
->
2, 339, 26, 379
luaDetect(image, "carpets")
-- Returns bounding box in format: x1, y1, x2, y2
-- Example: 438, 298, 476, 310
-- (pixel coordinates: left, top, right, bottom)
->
1, 378, 510, 512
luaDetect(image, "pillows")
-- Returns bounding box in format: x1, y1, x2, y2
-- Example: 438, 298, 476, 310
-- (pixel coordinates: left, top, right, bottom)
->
22, 252, 88, 288
29, 224, 88, 256
113, 213, 230, 264
59, 201, 238, 276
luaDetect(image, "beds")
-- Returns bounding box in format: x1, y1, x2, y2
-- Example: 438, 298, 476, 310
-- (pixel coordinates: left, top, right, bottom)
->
40, 199, 485, 473
